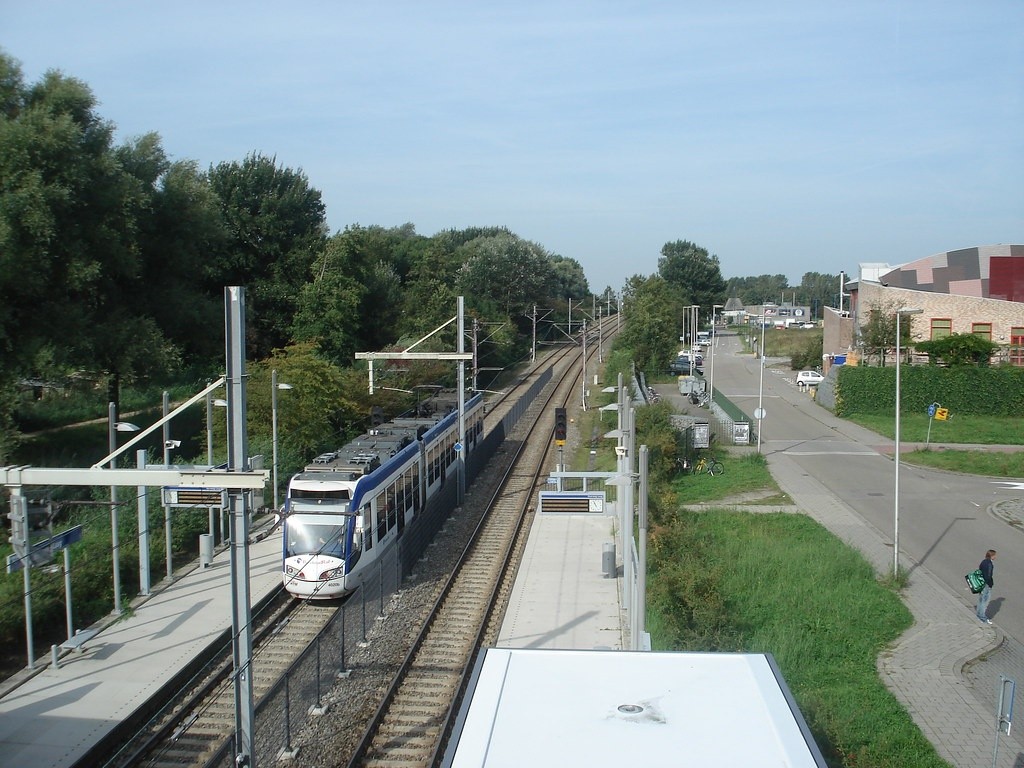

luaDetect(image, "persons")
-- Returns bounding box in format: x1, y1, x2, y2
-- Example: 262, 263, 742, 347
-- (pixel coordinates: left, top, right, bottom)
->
977, 550, 997, 625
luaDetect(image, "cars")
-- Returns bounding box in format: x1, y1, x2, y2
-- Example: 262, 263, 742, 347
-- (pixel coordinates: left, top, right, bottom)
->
668, 328, 716, 377
797, 371, 825, 386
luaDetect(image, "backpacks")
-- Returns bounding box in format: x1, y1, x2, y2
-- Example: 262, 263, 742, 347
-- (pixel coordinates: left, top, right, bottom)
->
965, 569, 986, 594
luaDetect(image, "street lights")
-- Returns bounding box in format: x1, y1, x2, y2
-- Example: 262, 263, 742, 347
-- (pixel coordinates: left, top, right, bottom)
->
683, 305, 700, 376
598, 373, 649, 652
894, 308, 924, 580
108, 402, 140, 611
272, 370, 293, 510
207, 382, 227, 550
757, 305, 778, 453
710, 305, 723, 402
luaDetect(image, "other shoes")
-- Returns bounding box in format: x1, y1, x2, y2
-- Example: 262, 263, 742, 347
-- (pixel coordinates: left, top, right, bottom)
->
987, 619, 992, 625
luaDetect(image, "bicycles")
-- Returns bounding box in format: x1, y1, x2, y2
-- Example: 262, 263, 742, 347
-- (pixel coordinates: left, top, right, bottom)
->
647, 387, 661, 404
677, 457, 724, 477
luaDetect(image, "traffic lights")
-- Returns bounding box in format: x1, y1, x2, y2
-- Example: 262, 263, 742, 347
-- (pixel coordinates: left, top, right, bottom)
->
555, 408, 566, 440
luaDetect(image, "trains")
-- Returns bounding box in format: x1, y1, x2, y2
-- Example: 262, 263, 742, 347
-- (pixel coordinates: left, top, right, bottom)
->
282, 388, 485, 599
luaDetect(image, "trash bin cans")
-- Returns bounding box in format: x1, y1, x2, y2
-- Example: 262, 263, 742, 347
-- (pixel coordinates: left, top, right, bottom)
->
678, 375, 705, 396
199, 534, 213, 566
602, 543, 617, 578
